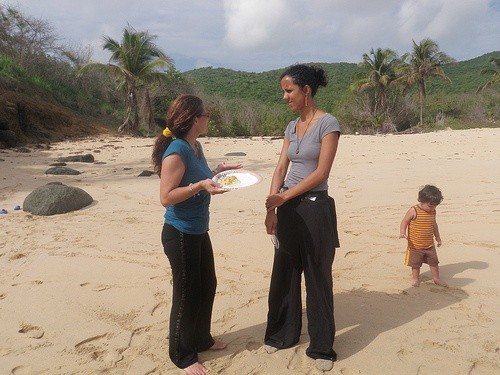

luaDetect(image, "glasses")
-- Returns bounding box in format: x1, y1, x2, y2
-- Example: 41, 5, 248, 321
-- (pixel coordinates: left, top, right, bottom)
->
200, 111, 211, 118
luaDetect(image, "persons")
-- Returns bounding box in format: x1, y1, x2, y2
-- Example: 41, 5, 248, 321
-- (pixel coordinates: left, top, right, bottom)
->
152, 93, 244, 375
398, 185, 449, 289
263, 63, 340, 371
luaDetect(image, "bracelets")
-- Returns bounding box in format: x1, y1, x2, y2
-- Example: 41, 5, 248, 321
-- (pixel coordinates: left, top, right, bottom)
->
189, 182, 201, 199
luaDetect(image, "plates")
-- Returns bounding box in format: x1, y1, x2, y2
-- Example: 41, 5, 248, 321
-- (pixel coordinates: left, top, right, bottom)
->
212, 169, 261, 190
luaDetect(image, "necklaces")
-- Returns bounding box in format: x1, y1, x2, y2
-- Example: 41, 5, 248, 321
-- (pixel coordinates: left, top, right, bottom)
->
295, 108, 319, 154
